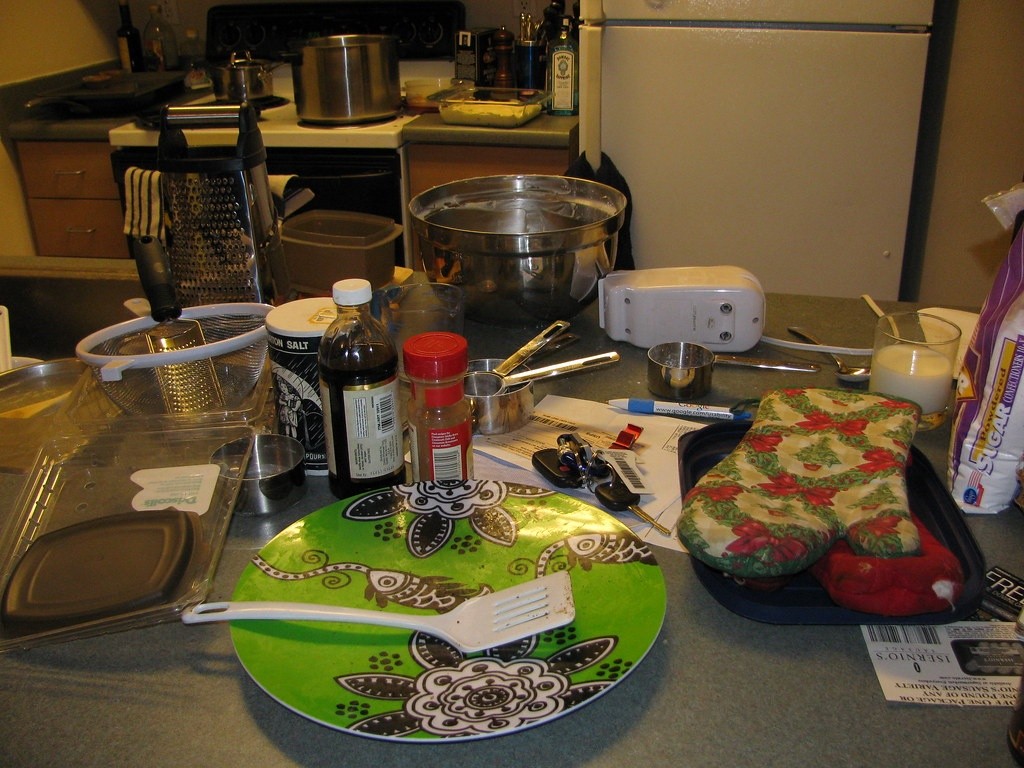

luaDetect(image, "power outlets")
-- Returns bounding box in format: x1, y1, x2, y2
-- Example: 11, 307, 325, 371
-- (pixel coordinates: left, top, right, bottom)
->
156, 0, 180, 25
513, 0, 537, 18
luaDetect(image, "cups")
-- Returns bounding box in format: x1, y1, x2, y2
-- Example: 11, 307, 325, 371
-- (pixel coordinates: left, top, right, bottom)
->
869, 313, 962, 431
514, 41, 546, 89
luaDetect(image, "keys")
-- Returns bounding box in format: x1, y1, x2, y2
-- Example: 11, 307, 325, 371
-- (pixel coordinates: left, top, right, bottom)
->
531, 432, 672, 535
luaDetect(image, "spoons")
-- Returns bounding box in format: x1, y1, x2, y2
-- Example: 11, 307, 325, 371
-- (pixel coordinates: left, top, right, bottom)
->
790, 326, 871, 382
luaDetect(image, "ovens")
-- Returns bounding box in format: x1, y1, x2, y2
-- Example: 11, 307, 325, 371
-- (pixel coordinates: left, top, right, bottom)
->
237, 147, 405, 266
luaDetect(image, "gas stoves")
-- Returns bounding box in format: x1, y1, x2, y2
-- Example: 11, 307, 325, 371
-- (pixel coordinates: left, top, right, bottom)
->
109, 0, 466, 148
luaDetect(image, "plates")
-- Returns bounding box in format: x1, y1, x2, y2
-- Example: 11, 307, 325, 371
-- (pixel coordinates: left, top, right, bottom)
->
229, 479, 667, 742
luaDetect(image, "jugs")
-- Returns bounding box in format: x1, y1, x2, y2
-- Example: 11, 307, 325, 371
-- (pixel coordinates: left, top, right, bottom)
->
380, 283, 465, 385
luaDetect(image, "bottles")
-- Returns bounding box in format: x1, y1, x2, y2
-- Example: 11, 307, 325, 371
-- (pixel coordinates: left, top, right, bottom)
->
547, 20, 579, 115
266, 297, 337, 476
115, 0, 141, 72
402, 330, 475, 482
143, 5, 177, 71
317, 279, 407, 499
181, 30, 202, 64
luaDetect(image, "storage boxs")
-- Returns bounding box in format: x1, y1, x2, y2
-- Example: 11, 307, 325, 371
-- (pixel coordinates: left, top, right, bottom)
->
279, 210, 403, 295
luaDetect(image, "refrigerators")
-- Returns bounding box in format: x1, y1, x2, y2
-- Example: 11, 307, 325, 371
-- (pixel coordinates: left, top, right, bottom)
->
577, 0, 932, 301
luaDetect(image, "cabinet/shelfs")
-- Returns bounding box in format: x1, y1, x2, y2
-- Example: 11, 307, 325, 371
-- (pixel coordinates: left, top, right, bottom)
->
1, 95, 141, 261
402, 105, 580, 272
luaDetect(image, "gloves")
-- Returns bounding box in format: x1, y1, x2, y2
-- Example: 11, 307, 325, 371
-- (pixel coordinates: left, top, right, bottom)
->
676, 388, 921, 578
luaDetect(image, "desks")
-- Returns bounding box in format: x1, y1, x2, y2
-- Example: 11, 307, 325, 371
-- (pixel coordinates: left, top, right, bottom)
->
0, 270, 1024, 768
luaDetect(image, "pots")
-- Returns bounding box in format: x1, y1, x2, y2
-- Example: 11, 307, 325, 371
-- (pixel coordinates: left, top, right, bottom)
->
211, 50, 267, 101
466, 320, 621, 434
280, 34, 402, 124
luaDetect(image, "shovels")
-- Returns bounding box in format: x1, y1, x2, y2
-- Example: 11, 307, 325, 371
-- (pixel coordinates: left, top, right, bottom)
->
133, 234, 225, 415
180, 570, 576, 654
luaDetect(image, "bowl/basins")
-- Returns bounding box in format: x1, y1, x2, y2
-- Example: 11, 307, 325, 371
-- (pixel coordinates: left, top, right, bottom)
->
209, 433, 305, 517
405, 79, 552, 125
647, 342, 715, 398
409, 174, 628, 326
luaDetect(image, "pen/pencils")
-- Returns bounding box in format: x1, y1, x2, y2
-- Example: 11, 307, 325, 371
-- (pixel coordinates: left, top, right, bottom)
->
606, 399, 751, 421
